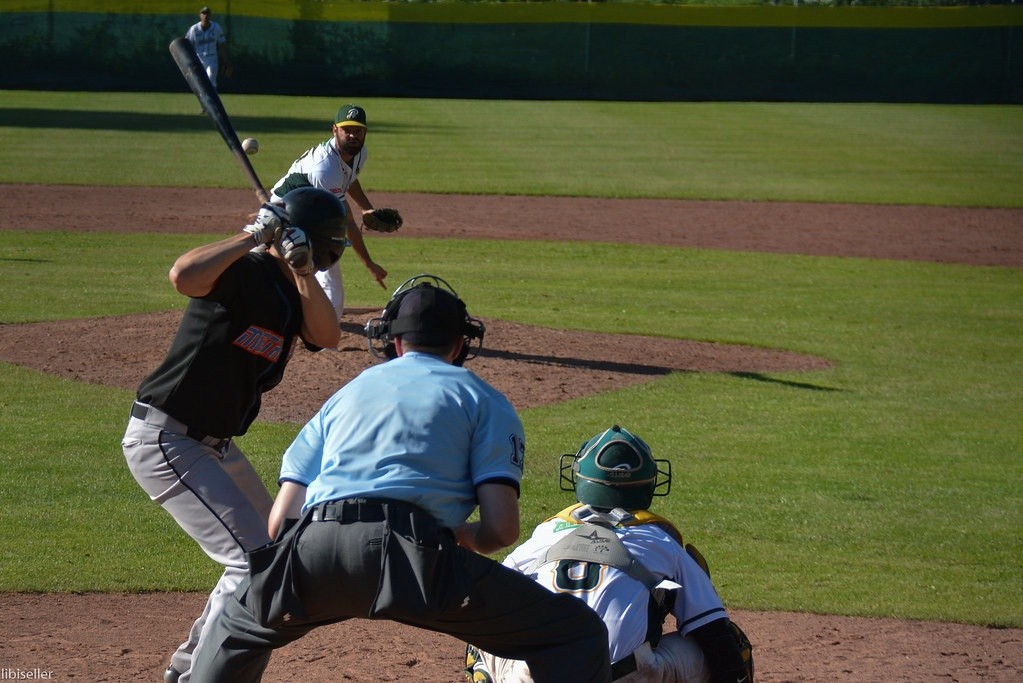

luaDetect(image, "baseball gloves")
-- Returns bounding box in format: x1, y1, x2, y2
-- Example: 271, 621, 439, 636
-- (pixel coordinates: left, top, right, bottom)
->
361, 208, 404, 233
220, 63, 233, 78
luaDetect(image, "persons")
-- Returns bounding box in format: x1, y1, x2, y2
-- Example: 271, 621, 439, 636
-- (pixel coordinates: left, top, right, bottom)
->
121, 188, 347, 683
184, 7, 225, 114
189, 273, 614, 683
249, 105, 404, 322
464, 425, 756, 683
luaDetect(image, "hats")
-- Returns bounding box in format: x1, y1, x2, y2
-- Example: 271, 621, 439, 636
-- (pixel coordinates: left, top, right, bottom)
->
335, 104, 367, 128
389, 285, 466, 346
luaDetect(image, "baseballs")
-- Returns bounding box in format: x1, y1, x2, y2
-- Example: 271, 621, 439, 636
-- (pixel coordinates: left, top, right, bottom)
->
242, 137, 259, 155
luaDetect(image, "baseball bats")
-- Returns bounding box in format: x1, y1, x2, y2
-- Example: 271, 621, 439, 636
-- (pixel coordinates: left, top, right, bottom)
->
169, 37, 307, 269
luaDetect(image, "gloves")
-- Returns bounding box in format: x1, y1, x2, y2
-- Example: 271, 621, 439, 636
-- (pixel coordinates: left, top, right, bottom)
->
274, 227, 314, 276
242, 202, 294, 247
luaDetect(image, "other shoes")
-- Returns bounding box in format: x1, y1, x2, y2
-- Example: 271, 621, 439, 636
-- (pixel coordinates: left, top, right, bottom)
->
463, 643, 493, 683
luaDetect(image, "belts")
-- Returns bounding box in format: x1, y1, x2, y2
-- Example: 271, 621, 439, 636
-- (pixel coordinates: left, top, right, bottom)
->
610, 652, 637, 681
309, 501, 438, 536
131, 403, 230, 451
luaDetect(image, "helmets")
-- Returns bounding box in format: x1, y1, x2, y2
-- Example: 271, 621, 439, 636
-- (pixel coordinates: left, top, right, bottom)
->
558, 424, 672, 510
282, 187, 348, 272
